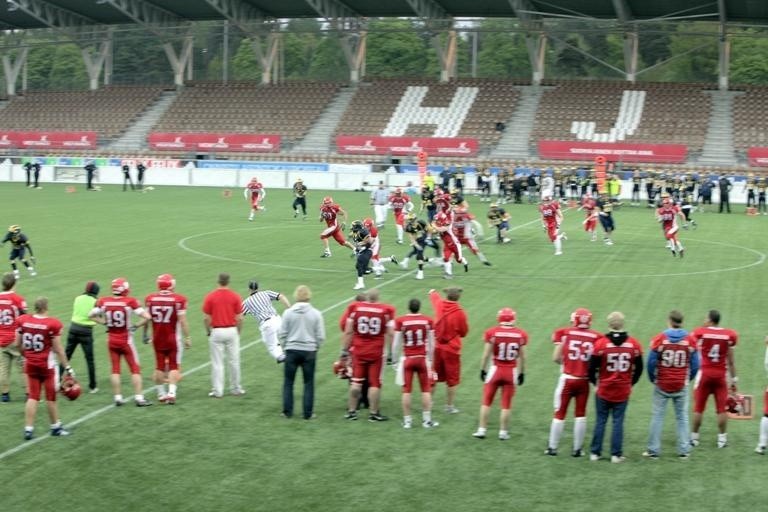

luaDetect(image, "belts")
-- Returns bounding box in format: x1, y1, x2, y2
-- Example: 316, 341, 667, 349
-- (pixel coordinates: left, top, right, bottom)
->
213, 326, 236, 328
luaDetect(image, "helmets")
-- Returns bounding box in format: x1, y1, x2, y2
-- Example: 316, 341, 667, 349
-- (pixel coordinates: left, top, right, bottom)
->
9, 225, 20, 237
158, 274, 174, 290
434, 187, 443, 195
726, 393, 745, 413
582, 194, 594, 201
496, 308, 516, 326
422, 186, 429, 192
600, 193, 607, 201
334, 360, 352, 379
454, 209, 468, 221
407, 214, 416, 222
489, 204, 498, 210
661, 192, 669, 199
662, 198, 673, 208
60, 376, 81, 401
542, 196, 554, 205
434, 213, 446, 225
323, 197, 332, 204
363, 219, 373, 228
111, 278, 129, 296
251, 178, 258, 183
296, 178, 303, 183
450, 188, 457, 196
86, 282, 99, 296
395, 188, 401, 195
351, 221, 362, 231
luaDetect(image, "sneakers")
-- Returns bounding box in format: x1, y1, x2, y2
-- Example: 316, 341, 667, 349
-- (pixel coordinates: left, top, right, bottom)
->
248, 216, 255, 222
429, 258, 434, 265
343, 409, 358, 420
367, 413, 387, 422
678, 453, 690, 459
690, 439, 700, 446
114, 395, 124, 405
590, 455, 601, 460
391, 255, 397, 264
611, 456, 625, 463
231, 389, 245, 395
28, 268, 37, 277
573, 308, 592, 328
498, 432, 510, 440
671, 250, 676, 256
294, 212, 299, 218
159, 395, 169, 404
90, 387, 98, 393
353, 283, 365, 290
604, 238, 609, 241
472, 428, 486, 438
571, 450, 586, 457
503, 238, 511, 244
415, 271, 423, 279
642, 451, 660, 460
51, 427, 71, 436
136, 398, 152, 406
464, 262, 468, 272
562, 232, 567, 240
606, 241, 614, 245
350, 248, 357, 258
755, 445, 765, 454
261, 206, 265, 214
281, 411, 293, 418
421, 420, 439, 428
320, 252, 331, 258
396, 240, 403, 245
2, 393, 10, 401
592, 235, 596, 241
306, 413, 318, 419
303, 214, 307, 220
401, 420, 413, 428
554, 251, 562, 255
25, 430, 31, 439
544, 447, 558, 456
168, 394, 175, 404
375, 273, 381, 276
482, 261, 491, 266
717, 441, 727, 448
679, 248, 683, 255
208, 391, 224, 397
380, 265, 388, 273
399, 261, 407, 268
446, 407, 459, 414
442, 273, 452, 279
14, 272, 19, 279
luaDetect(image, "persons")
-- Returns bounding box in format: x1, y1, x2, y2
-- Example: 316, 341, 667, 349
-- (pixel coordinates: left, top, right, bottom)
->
370, 181, 389, 229
393, 300, 440, 428
496, 167, 601, 206
630, 170, 641, 206
359, 219, 398, 277
425, 171, 434, 191
292, 176, 307, 219
744, 172, 756, 208
436, 188, 469, 212
472, 307, 529, 439
319, 197, 356, 257
588, 311, 643, 464
122, 163, 134, 190
244, 177, 266, 221
340, 294, 366, 331
241, 281, 291, 364
543, 308, 603, 457
85, 160, 96, 188
399, 210, 441, 280
59, 281, 100, 394
428, 287, 469, 413
16, 296, 76, 440
597, 194, 624, 245
278, 285, 325, 419
203, 272, 246, 398
137, 163, 146, 188
389, 188, 412, 244
487, 204, 511, 243
689, 310, 738, 448
718, 177, 733, 213
657, 198, 687, 257
0, 225, 36, 280
755, 335, 768, 453
539, 194, 568, 255
645, 169, 715, 210
439, 165, 466, 196
88, 277, 152, 406
421, 184, 436, 221
340, 289, 394, 422
757, 177, 767, 215
425, 212, 468, 279
641, 311, 691, 459
31, 162, 41, 187
476, 162, 492, 201
578, 194, 600, 242
23, 161, 33, 186
348, 221, 373, 290
0, 273, 30, 401
141, 274, 192, 404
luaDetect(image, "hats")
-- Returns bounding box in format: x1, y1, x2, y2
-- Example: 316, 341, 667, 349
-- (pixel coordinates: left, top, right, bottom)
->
248, 281, 258, 290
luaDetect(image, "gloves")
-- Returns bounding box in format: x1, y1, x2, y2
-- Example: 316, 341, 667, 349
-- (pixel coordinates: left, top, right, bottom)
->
323, 212, 328, 218
340, 223, 346, 231
412, 242, 417, 247
543, 224, 547, 233
356, 246, 364, 251
184, 337, 192, 348
339, 348, 348, 358
730, 377, 738, 392
65, 366, 74, 376
665, 215, 671, 219
556, 223, 560, 228
683, 224, 688, 229
479, 370, 487, 381
142, 335, 151, 345
425, 237, 431, 243
129, 325, 137, 333
386, 355, 394, 365
518, 373, 525, 386
